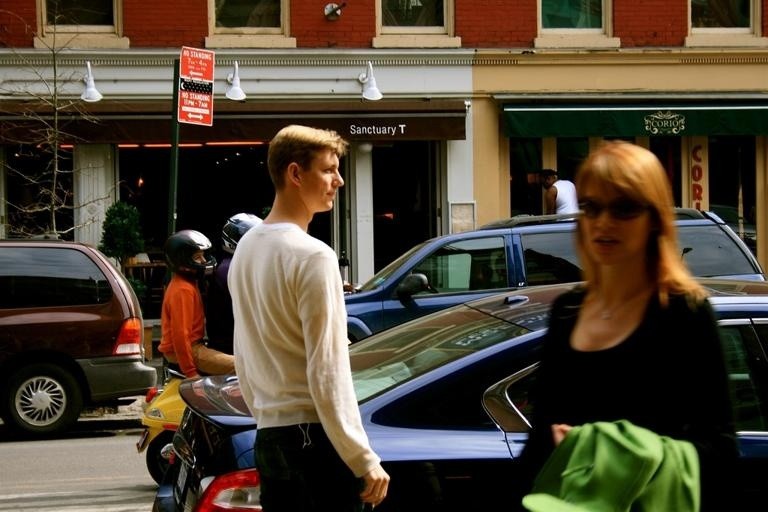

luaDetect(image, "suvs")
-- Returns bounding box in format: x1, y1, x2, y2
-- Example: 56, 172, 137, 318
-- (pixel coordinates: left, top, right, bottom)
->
0, 243, 157, 436
342, 205, 766, 349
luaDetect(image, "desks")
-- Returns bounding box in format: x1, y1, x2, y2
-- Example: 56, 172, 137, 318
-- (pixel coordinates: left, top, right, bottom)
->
121, 260, 168, 319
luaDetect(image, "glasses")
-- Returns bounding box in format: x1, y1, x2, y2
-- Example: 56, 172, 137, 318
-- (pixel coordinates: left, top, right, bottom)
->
577, 197, 648, 221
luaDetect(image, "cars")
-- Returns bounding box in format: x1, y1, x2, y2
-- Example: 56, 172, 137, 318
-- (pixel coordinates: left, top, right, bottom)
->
151, 273, 767, 510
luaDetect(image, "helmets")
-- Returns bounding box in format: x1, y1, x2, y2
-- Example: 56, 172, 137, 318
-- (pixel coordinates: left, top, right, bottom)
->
222, 213, 264, 254
164, 230, 217, 276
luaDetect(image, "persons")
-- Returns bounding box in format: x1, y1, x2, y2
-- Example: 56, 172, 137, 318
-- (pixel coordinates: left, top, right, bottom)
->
539, 166, 580, 217
223, 122, 392, 511
204, 210, 267, 355
512, 140, 743, 512
154, 227, 235, 380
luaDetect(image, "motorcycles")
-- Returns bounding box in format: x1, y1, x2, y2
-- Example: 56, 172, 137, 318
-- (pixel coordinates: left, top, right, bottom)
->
133, 366, 192, 482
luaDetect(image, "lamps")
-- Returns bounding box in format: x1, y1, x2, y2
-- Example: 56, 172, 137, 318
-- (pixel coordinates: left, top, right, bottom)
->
80, 61, 103, 103
225, 61, 246, 101
358, 60, 384, 101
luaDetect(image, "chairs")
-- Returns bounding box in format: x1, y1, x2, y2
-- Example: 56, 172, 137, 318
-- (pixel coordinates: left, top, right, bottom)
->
479, 266, 496, 289
